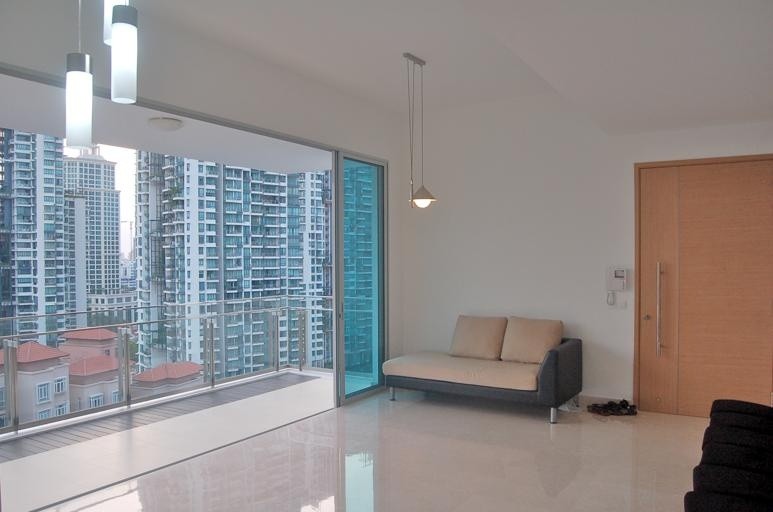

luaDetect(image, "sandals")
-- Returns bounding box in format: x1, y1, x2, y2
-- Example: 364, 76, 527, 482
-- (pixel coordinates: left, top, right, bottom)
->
588, 400, 637, 416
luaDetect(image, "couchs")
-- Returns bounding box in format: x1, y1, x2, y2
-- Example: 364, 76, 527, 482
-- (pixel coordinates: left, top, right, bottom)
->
383, 338, 582, 423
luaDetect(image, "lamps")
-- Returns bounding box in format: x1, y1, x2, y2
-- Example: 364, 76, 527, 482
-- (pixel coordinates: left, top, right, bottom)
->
65, 0, 140, 149
403, 52, 437, 208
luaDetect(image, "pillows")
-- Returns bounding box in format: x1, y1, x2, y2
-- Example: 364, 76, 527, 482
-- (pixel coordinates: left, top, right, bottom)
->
500, 316, 564, 363
450, 314, 507, 360
